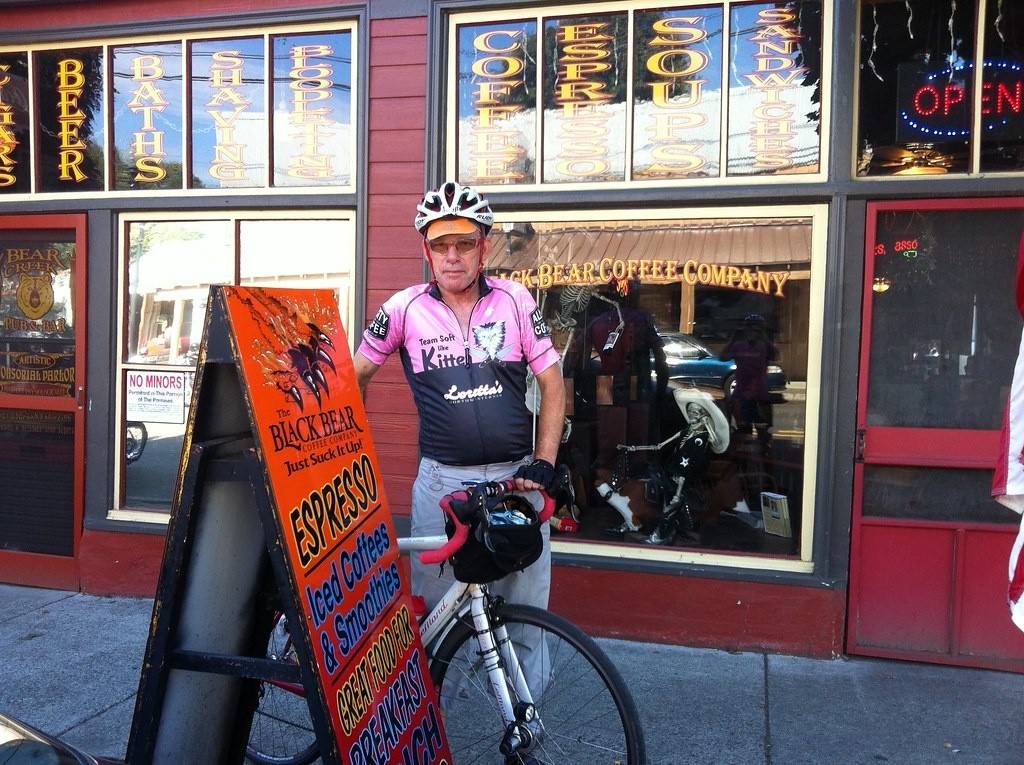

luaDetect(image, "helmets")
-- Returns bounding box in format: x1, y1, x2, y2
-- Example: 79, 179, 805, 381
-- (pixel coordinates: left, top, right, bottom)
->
615, 270, 642, 292
744, 313, 766, 324
414, 181, 494, 241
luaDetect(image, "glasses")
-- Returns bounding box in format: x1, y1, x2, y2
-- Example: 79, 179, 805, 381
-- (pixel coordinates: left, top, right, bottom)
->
424, 228, 484, 255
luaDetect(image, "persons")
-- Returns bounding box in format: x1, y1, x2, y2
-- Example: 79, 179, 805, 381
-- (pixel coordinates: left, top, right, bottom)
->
355, 181, 566, 702
574, 269, 670, 508
719, 315, 781, 452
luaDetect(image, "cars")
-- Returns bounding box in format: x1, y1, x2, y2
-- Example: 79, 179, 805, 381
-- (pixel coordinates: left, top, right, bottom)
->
588, 330, 788, 402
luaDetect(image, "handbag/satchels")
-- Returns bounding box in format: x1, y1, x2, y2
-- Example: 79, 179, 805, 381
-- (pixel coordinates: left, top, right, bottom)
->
439, 481, 543, 583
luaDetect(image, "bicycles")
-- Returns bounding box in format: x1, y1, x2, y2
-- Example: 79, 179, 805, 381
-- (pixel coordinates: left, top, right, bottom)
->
244, 481, 646, 764
